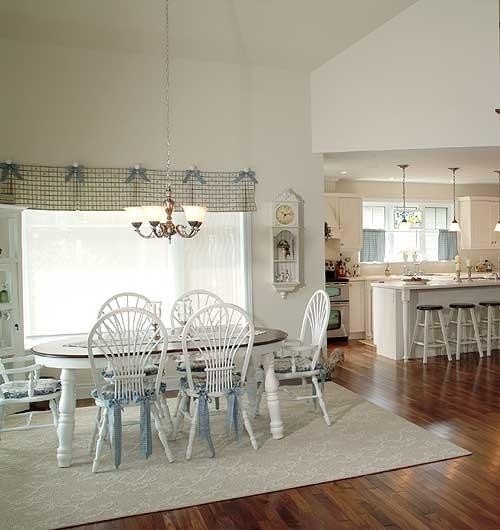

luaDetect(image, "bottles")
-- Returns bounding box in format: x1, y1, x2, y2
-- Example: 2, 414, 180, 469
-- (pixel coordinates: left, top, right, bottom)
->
335, 260, 360, 278
275, 270, 290, 283
483, 259, 492, 272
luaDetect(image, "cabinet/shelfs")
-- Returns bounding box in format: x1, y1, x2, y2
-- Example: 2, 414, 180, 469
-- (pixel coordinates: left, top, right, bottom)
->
0, 204, 30, 381
349, 278, 371, 341
458, 195, 499, 250
322, 194, 365, 249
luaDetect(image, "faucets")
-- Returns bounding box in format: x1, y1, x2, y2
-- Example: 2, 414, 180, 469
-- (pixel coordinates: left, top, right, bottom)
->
413, 259, 427, 274
488, 272, 498, 280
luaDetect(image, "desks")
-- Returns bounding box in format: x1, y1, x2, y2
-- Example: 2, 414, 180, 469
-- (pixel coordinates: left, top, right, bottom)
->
31, 326, 290, 468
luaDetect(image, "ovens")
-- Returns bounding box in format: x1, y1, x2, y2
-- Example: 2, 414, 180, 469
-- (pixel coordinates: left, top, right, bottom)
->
326, 283, 350, 342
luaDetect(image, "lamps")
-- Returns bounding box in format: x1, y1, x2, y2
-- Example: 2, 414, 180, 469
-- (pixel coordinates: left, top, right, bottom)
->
447, 167, 462, 232
126, 0, 208, 241
395, 163, 411, 231
494, 169, 500, 231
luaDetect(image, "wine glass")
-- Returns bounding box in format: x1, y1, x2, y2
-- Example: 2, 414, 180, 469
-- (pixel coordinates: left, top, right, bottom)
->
146, 302, 162, 341
179, 299, 198, 338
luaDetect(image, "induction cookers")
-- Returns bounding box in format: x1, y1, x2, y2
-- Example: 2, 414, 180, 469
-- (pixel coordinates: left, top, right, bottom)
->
325, 278, 350, 284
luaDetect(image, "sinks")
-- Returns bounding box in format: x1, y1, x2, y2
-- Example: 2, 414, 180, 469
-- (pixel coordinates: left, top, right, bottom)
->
403, 273, 451, 277
453, 277, 495, 280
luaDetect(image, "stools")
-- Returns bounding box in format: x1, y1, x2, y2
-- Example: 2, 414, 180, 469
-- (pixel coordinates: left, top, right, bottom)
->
476, 301, 500, 358
445, 302, 485, 360
407, 304, 452, 365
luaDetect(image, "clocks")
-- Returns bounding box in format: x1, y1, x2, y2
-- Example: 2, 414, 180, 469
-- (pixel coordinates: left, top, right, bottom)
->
271, 189, 303, 301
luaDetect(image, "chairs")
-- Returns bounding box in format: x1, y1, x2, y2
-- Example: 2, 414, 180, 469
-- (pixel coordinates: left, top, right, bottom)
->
96, 292, 172, 427
0, 354, 63, 429
174, 301, 260, 460
253, 290, 346, 427
88, 308, 175, 473
170, 290, 231, 415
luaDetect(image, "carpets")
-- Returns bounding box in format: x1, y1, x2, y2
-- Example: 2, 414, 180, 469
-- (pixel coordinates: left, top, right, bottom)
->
0, 381, 472, 529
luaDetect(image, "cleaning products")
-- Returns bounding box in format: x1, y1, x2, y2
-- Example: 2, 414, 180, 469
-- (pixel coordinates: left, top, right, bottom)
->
384, 260, 392, 277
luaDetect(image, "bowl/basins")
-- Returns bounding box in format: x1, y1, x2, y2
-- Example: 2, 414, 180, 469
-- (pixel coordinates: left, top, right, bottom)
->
326, 271, 336, 282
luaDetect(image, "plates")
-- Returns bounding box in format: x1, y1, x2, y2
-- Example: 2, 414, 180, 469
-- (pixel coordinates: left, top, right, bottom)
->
402, 279, 431, 284
